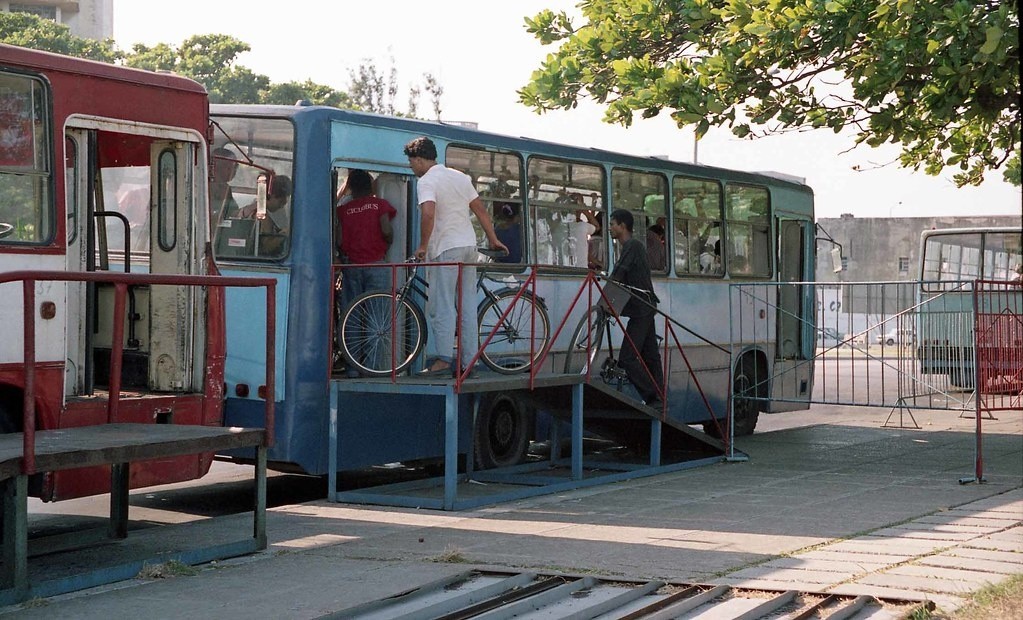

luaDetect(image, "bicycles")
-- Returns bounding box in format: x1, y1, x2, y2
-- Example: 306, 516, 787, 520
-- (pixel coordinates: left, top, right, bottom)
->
563, 270, 671, 394
342, 249, 553, 371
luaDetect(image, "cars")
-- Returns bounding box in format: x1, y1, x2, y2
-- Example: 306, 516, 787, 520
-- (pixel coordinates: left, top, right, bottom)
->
813, 325, 848, 350
877, 328, 916, 347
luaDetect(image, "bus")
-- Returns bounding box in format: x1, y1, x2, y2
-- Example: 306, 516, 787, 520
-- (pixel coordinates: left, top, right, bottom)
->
913, 224, 1022, 397
0, 40, 275, 547
204, 99, 818, 474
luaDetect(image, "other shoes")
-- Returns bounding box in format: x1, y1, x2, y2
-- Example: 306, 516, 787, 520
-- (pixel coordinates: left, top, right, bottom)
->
347, 365, 360, 378
413, 363, 453, 380
452, 367, 479, 379
647, 400, 669, 412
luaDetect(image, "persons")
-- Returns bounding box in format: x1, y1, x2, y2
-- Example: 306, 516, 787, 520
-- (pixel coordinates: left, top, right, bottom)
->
103, 148, 397, 378
606, 209, 670, 413
403, 136, 509, 379
471, 169, 748, 273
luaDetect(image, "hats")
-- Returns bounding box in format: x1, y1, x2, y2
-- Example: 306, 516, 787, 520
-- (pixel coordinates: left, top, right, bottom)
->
502, 201, 522, 218
